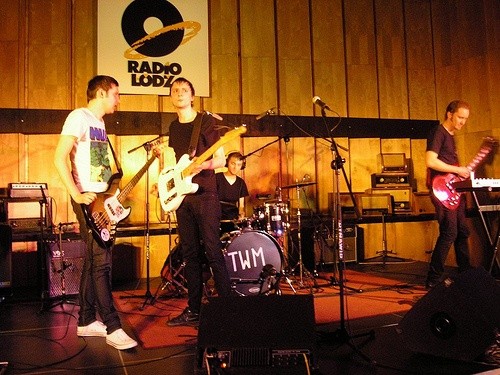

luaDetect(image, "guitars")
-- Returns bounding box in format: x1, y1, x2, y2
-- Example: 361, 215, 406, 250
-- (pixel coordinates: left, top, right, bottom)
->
158, 124, 247, 212
432, 138, 495, 210
79, 149, 159, 248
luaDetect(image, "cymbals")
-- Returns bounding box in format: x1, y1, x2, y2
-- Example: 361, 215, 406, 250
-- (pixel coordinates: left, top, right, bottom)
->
281, 182, 319, 190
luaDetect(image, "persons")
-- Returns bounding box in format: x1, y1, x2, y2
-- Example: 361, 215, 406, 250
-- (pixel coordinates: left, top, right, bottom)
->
166, 77, 232, 326
215, 151, 249, 239
54, 75, 137, 350
426, 100, 476, 290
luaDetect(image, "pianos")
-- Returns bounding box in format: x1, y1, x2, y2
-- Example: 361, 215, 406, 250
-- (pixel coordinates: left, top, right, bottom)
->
449, 178, 500, 277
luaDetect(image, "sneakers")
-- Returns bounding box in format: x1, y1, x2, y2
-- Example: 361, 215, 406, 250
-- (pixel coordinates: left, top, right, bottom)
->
76, 320, 107, 337
106, 328, 138, 349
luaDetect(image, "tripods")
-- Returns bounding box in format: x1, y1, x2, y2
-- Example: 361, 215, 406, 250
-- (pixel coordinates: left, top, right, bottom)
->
119, 133, 188, 312
37, 231, 81, 316
272, 112, 376, 369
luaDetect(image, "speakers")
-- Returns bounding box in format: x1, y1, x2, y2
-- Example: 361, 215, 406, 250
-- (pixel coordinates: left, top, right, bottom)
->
194, 292, 319, 375
388, 265, 500, 362
0, 224, 11, 287
36, 233, 82, 300
312, 225, 357, 262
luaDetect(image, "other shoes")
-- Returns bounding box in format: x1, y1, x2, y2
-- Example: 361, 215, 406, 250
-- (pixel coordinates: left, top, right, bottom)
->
167, 308, 200, 325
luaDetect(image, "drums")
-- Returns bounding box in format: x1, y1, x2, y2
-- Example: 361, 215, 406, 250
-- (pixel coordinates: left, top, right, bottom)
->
253, 204, 289, 236
224, 231, 283, 297
218, 220, 247, 241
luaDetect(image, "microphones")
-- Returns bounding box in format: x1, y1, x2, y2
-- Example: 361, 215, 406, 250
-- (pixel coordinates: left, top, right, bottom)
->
313, 96, 330, 110
256, 108, 274, 120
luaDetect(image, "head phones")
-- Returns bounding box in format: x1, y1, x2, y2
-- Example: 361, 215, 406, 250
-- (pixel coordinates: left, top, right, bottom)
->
223, 150, 246, 170
